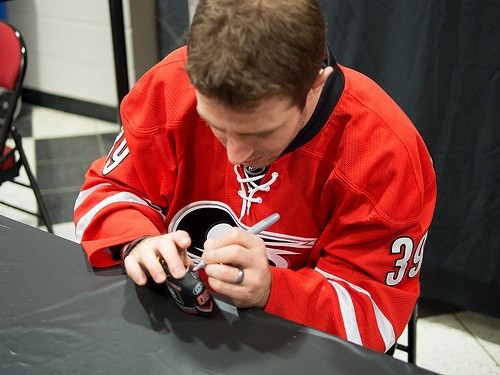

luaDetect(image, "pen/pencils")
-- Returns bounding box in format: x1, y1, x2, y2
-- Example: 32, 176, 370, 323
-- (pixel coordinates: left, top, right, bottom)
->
192, 213, 281, 272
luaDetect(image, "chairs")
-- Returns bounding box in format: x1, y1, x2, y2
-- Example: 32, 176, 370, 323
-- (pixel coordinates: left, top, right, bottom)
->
0, 20, 55, 234
391, 301, 418, 369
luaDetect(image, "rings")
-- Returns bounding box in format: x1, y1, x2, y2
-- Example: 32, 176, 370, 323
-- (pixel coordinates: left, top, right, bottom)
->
235, 267, 244, 284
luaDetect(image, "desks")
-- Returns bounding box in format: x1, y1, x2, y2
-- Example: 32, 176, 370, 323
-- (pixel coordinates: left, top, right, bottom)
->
0, 216, 442, 375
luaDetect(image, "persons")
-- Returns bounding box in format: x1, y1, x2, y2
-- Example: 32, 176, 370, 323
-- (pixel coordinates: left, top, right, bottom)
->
72, 0, 438, 354
